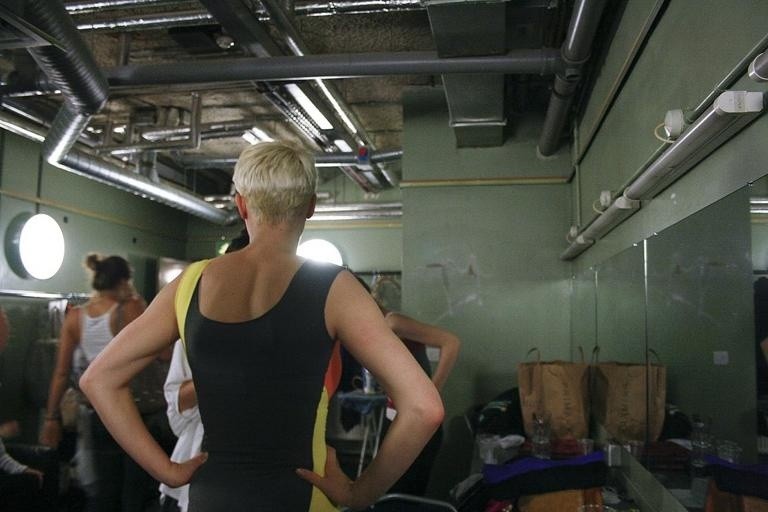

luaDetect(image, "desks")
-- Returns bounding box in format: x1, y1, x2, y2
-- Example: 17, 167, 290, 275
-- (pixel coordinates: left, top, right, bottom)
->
337, 392, 389, 480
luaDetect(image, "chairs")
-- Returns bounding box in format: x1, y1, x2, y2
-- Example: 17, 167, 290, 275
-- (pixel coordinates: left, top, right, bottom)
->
369, 493, 456, 510
6, 444, 59, 511
465, 401, 483, 436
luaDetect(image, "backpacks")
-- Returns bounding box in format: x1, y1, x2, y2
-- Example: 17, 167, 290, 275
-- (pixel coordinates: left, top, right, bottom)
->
455, 451, 609, 512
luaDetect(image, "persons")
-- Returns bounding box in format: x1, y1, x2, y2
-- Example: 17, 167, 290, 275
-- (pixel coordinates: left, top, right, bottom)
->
40, 251, 173, 511
159, 338, 204, 511
349, 277, 461, 512
78, 141, 445, 511
0, 309, 44, 512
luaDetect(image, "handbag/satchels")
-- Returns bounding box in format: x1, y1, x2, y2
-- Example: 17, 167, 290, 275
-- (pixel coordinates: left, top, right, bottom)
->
642, 440, 768, 500
517, 346, 591, 439
591, 346, 666, 446
68, 365, 168, 415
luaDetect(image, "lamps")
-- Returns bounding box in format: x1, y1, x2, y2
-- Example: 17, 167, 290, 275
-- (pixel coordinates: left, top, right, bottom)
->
560, 235, 593, 260
582, 196, 641, 241
623, 90, 768, 201
748, 48, 767, 81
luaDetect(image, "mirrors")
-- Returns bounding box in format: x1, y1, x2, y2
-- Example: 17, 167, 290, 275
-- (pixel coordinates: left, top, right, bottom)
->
570, 173, 767, 511
